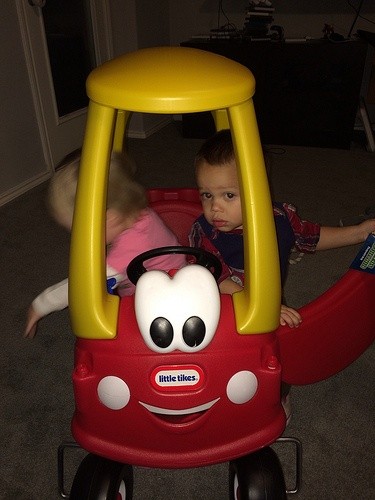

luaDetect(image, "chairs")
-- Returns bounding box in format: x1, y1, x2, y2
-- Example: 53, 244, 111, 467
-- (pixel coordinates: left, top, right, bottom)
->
139, 202, 205, 264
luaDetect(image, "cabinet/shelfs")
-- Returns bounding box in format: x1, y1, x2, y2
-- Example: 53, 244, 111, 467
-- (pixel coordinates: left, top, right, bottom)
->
175, 38, 367, 149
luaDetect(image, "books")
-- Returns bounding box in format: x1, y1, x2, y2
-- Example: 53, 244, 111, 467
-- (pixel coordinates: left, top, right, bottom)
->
209, 0, 307, 42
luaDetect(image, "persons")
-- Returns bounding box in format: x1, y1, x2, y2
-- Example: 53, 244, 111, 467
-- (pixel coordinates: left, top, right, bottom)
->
21, 153, 188, 341
187, 129, 375, 427
